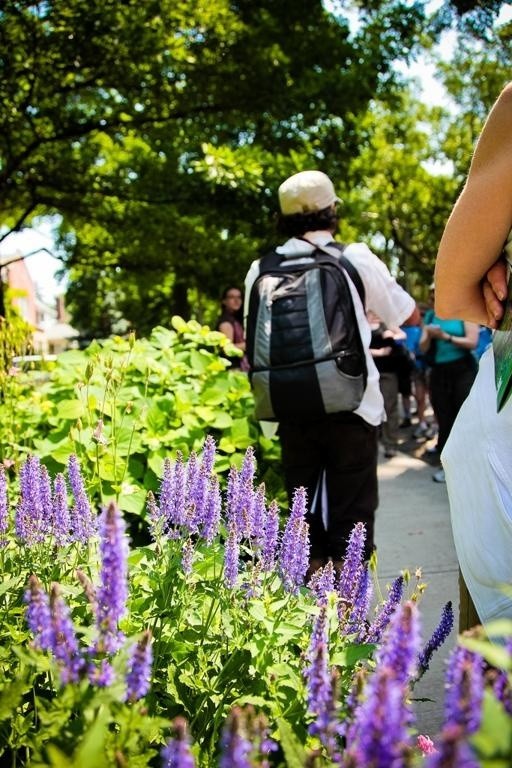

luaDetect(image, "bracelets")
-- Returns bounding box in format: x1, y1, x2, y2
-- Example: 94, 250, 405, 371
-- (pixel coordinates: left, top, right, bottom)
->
447, 334, 455, 344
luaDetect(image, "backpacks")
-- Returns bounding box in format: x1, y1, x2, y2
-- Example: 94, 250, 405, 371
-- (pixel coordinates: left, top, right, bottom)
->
243, 242, 372, 423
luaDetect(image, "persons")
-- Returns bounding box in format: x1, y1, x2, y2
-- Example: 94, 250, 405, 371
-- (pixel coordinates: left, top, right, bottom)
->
361, 281, 440, 460
431, 78, 512, 660
239, 169, 423, 587
214, 285, 255, 373
417, 282, 484, 484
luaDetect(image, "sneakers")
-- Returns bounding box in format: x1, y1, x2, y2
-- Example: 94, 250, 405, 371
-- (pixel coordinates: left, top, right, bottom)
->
384, 449, 397, 457
431, 470, 445, 482
398, 417, 438, 439
427, 445, 439, 454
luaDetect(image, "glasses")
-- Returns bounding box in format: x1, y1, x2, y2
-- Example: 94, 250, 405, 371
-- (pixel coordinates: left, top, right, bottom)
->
227, 296, 241, 300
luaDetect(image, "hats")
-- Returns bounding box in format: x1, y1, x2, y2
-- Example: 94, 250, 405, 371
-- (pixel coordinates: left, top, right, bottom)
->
278, 170, 343, 216
